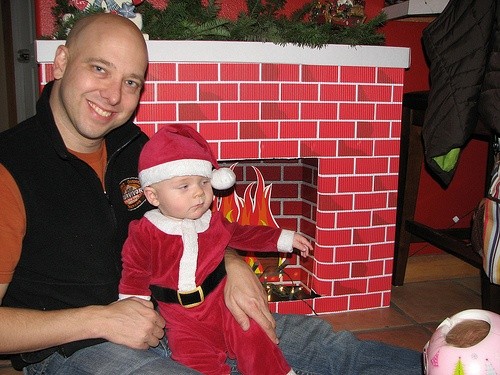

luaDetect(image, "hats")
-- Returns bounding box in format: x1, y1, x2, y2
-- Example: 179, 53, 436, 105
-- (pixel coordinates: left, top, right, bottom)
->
137, 123, 237, 191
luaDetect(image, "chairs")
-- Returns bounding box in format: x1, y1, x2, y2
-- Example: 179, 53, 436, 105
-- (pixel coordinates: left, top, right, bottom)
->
393, 0, 500, 315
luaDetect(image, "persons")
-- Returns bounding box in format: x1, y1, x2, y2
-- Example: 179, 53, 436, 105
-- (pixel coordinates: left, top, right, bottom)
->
117, 124, 313, 375
0, 12, 425, 375
